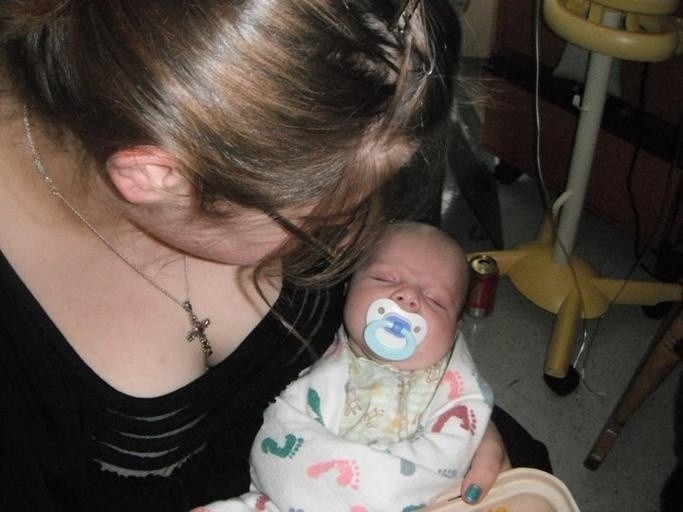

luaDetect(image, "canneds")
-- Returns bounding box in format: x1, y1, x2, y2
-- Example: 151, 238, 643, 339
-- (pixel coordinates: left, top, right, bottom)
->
470, 251, 500, 318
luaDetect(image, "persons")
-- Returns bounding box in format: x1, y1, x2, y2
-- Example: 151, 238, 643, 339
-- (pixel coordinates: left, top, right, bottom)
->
191, 221, 496, 512
0, 0, 514, 512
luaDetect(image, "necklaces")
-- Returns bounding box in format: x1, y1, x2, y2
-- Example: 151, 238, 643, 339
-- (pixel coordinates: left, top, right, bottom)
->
22, 103, 214, 359
